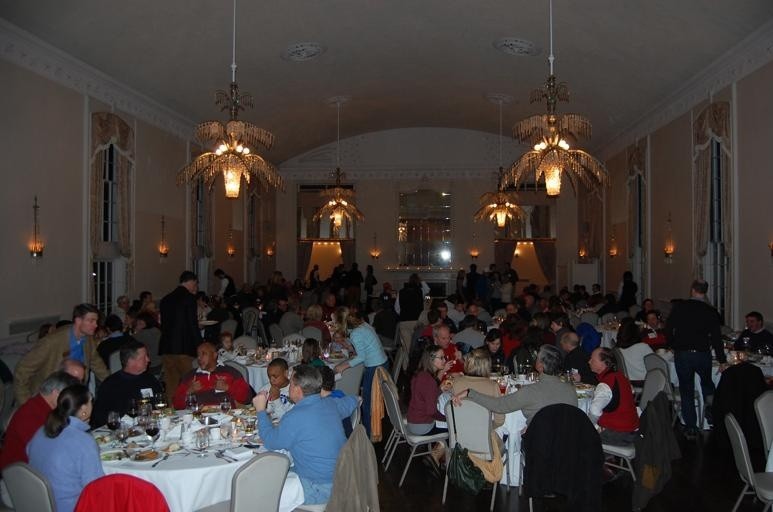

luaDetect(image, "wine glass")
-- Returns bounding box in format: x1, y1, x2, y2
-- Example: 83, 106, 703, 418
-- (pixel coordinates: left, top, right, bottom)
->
93, 389, 276, 464
232, 334, 355, 367
601, 314, 651, 330
491, 310, 504, 324
490, 360, 593, 396
717, 332, 772, 368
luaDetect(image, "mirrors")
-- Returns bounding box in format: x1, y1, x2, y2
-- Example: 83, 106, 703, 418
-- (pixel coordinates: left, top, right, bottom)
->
392, 184, 456, 268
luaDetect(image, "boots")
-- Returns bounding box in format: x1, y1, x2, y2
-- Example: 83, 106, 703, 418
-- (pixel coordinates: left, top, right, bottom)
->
422, 441, 446, 481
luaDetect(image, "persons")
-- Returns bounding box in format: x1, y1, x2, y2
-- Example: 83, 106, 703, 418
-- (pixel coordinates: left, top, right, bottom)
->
395, 258, 773, 512
3, 258, 401, 511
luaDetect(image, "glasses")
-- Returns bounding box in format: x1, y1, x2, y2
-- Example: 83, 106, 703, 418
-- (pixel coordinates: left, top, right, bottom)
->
434, 355, 446, 360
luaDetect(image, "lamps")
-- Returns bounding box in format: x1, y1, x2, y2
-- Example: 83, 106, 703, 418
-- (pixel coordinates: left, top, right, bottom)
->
501, 0, 611, 197
160, 215, 167, 258
31, 195, 42, 257
473, 92, 529, 228
312, 96, 365, 234
176, 0, 287, 200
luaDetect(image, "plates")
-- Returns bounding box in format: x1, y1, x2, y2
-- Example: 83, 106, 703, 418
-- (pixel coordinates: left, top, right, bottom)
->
199, 319, 218, 325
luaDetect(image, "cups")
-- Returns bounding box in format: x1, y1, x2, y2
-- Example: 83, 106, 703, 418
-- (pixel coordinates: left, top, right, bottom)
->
446, 354, 456, 362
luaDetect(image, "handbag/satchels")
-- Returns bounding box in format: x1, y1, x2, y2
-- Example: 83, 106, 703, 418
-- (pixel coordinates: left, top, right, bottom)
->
446, 441, 486, 499
